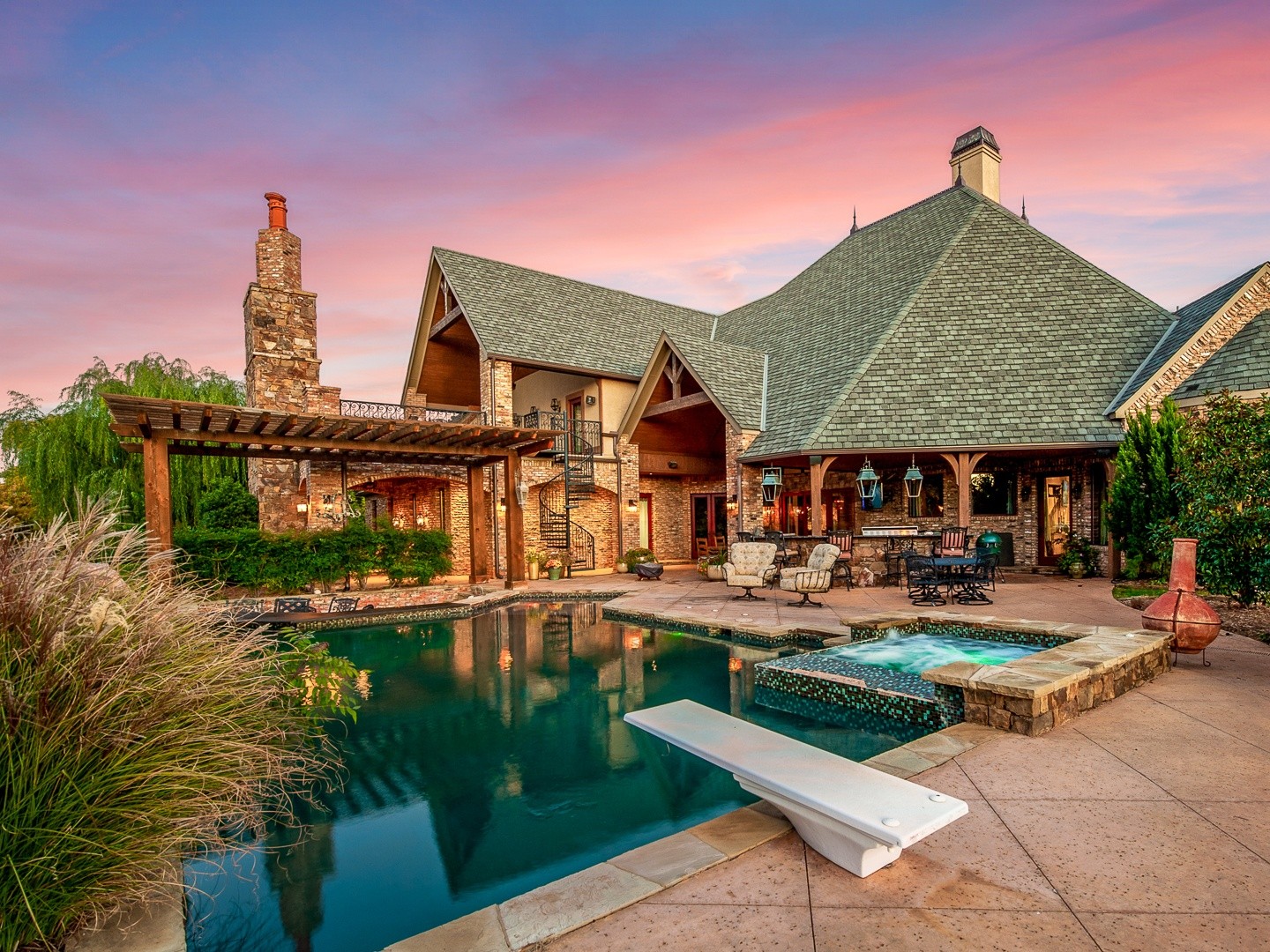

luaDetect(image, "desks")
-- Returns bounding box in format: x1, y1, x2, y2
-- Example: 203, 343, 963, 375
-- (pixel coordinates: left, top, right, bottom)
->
920, 557, 987, 600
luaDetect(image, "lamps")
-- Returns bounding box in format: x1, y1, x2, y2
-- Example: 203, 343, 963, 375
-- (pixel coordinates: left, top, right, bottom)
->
297, 495, 310, 513
392, 513, 405, 529
1072, 483, 1083, 500
320, 494, 336, 511
759, 460, 784, 502
511, 413, 521, 425
551, 398, 561, 413
415, 512, 429, 527
529, 406, 540, 419
726, 494, 738, 510
585, 395, 596, 405
903, 453, 923, 497
855, 454, 881, 498
515, 471, 530, 508
1020, 485, 1033, 502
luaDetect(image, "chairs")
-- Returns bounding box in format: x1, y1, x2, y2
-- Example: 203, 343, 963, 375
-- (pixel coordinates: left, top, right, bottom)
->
714, 535, 728, 555
948, 551, 999, 607
736, 532, 756, 542
826, 529, 855, 592
721, 542, 778, 602
779, 543, 841, 608
900, 548, 942, 600
931, 525, 970, 576
274, 597, 312, 614
952, 548, 1001, 600
327, 597, 361, 613
225, 598, 266, 613
696, 537, 721, 563
763, 530, 803, 590
881, 534, 919, 591
906, 554, 948, 607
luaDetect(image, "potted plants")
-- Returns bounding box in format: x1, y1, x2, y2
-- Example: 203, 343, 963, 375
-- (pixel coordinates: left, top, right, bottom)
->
1055, 530, 1102, 579
626, 547, 654, 561
615, 556, 628, 574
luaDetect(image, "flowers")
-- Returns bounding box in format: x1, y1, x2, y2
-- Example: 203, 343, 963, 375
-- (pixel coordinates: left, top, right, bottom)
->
544, 559, 562, 569
523, 547, 547, 564
697, 551, 728, 577
549, 549, 577, 567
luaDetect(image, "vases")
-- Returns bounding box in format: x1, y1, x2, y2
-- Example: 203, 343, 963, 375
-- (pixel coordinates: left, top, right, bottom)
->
707, 564, 726, 582
547, 567, 562, 580
528, 561, 539, 580
558, 566, 565, 578
